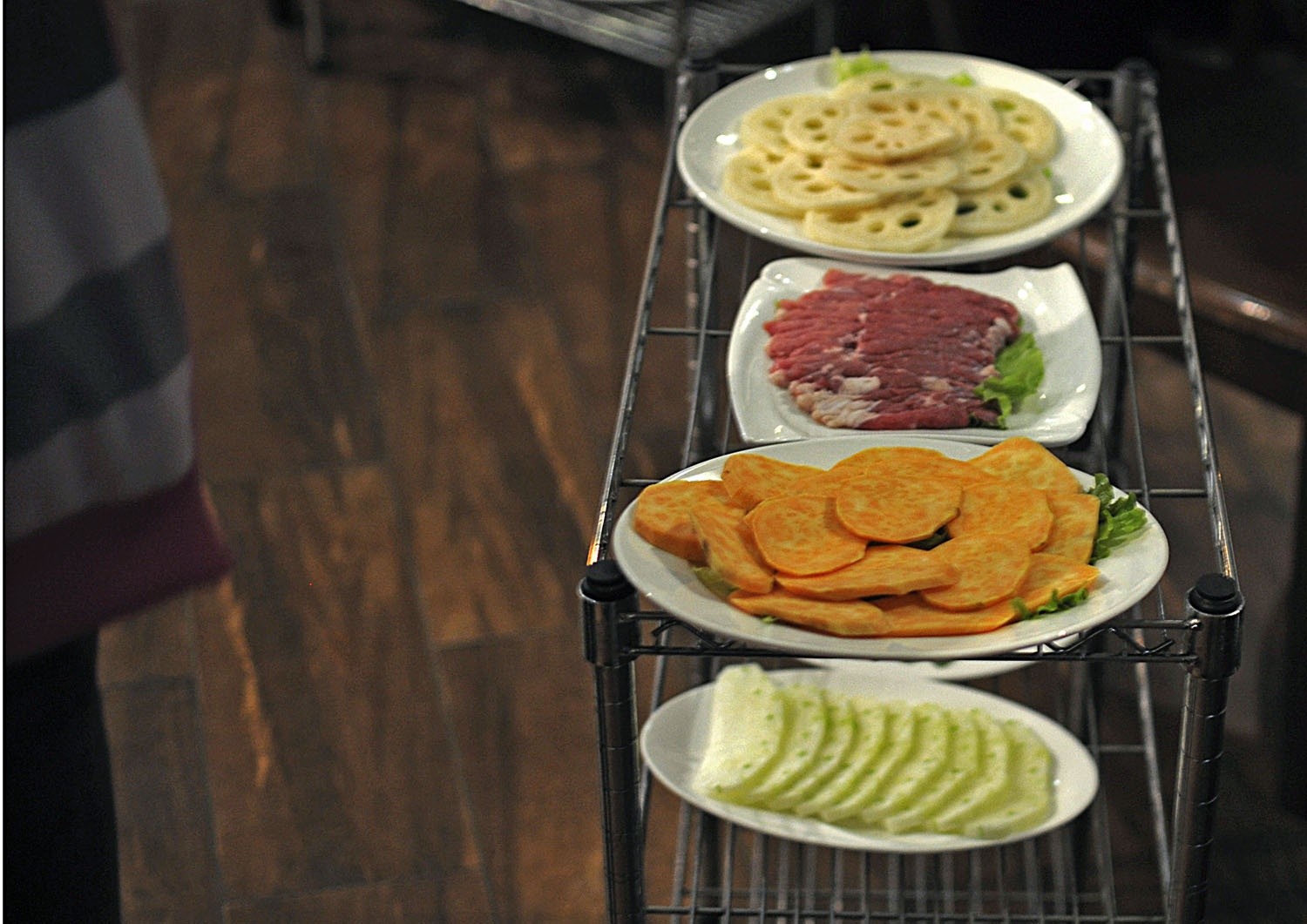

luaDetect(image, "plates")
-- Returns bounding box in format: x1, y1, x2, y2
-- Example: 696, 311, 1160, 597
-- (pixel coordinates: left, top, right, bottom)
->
675, 48, 1126, 264
727, 251, 1108, 447
637, 661, 1100, 854
612, 433, 1171, 657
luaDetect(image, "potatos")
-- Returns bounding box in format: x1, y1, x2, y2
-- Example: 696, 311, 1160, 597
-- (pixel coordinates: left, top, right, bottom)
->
635, 433, 1100, 633
